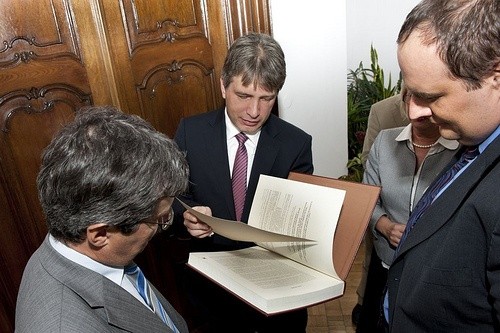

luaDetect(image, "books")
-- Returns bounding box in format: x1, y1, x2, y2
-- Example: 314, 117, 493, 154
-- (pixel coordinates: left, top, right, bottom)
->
187, 171, 383, 317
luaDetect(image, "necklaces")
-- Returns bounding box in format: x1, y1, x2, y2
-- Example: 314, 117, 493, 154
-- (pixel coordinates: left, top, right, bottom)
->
410, 142, 434, 148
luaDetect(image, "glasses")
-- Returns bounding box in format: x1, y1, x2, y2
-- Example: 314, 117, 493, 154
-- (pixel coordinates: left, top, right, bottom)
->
403, 92, 411, 104
140, 206, 175, 230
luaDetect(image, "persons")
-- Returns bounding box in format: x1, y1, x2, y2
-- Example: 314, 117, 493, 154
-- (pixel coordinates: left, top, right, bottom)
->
161, 32, 314, 333
355, 78, 412, 309
16, 106, 190, 333
360, 79, 463, 333
379, 0, 500, 333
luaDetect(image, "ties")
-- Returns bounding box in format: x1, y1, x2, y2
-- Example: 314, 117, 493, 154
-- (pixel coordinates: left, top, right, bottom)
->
392, 144, 480, 263
230, 132, 248, 222
124, 260, 181, 333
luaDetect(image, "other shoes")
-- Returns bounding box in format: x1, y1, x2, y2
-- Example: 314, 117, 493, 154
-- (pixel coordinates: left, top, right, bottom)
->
351, 303, 362, 326
